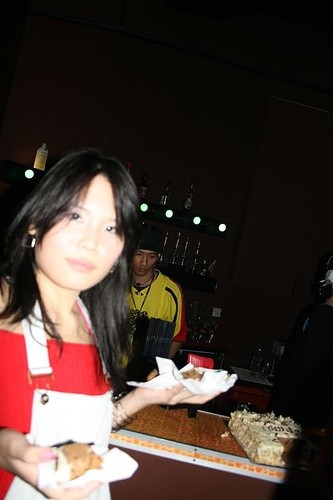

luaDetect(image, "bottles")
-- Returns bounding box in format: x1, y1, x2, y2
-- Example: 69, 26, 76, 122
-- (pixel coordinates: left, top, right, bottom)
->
183, 300, 222, 345
124, 160, 197, 216
152, 230, 208, 277
32, 136, 72, 172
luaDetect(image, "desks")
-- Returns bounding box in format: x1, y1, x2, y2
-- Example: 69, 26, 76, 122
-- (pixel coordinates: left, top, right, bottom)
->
109, 404, 285, 500
217, 366, 273, 413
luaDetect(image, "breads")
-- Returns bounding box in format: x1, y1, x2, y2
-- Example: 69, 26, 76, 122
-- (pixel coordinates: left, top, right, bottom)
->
61, 443, 103, 480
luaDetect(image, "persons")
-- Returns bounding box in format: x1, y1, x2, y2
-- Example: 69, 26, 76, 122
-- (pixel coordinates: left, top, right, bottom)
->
0, 149, 229, 500
267, 256, 333, 500
107, 227, 186, 394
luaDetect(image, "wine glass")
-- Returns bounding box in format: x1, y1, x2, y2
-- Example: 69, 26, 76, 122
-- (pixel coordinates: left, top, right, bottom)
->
249, 340, 285, 381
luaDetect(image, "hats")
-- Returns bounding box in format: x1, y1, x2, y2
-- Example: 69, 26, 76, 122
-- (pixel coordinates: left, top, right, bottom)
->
133, 224, 163, 253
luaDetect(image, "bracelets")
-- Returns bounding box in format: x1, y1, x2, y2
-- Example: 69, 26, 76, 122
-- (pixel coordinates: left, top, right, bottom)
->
111, 401, 136, 433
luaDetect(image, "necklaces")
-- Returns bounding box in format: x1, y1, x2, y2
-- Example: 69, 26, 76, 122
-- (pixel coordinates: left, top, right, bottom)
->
127, 274, 157, 310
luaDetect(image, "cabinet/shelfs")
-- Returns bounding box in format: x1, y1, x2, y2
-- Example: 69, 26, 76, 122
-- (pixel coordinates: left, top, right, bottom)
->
0, 158, 230, 356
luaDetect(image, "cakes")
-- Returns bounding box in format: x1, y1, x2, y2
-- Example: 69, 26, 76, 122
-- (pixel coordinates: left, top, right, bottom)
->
228, 408, 304, 467
